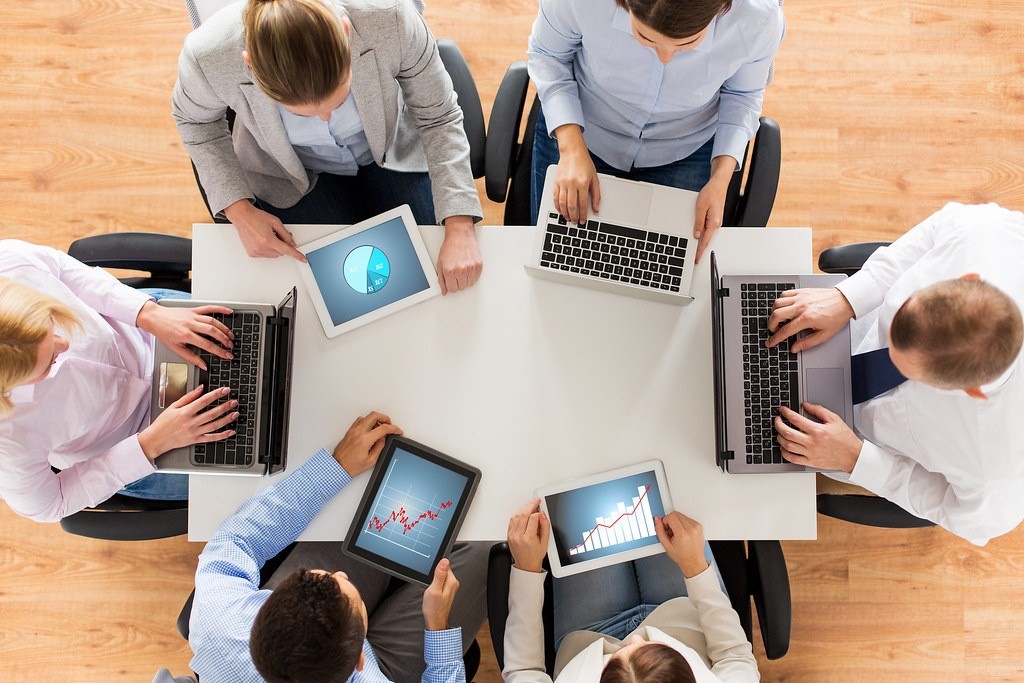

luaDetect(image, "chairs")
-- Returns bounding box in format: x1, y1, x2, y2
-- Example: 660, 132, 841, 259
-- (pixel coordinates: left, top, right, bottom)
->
174, 542, 482, 683
58, 231, 191, 540
486, 537, 792, 672
818, 239, 942, 531
484, 61, 782, 228
186, 0, 485, 225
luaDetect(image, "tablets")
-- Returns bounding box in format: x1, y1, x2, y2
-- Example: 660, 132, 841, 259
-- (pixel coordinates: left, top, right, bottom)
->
533, 459, 675, 579
342, 431, 483, 588
291, 204, 442, 340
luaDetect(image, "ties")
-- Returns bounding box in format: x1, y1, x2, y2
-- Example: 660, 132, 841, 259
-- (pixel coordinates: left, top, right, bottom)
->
851, 348, 907, 406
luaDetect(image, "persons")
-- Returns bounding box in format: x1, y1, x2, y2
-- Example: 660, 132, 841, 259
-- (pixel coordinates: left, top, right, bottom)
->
524, 0, 787, 266
0, 239, 240, 524
765, 202, 1024, 549
188, 412, 490, 683
170, 0, 484, 297
500, 496, 761, 683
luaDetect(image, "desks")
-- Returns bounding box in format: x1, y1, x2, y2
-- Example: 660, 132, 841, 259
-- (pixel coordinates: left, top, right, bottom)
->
187, 222, 819, 542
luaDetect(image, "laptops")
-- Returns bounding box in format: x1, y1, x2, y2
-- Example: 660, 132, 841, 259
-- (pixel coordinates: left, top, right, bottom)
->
522, 164, 702, 308
708, 249, 854, 475
149, 285, 299, 477
